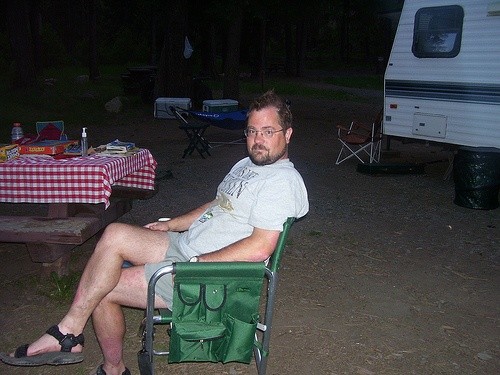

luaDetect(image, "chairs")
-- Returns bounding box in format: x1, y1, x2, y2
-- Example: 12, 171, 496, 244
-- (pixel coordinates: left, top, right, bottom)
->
137, 217, 295, 375
335, 104, 383, 166
168, 105, 212, 159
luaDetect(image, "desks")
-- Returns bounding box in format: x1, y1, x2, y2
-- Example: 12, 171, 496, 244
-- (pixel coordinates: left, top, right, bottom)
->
0, 148, 158, 279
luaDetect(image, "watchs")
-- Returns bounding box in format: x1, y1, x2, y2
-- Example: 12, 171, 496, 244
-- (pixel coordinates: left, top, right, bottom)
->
189, 256, 198, 262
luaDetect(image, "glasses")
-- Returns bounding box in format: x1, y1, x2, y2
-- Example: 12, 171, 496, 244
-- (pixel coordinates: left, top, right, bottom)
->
243, 127, 284, 138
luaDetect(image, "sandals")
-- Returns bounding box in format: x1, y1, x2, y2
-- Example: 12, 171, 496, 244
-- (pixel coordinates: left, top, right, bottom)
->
96, 364, 131, 375
0, 325, 84, 366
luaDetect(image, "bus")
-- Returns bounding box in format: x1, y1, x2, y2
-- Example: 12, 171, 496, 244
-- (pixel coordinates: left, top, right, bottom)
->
381, 0, 500, 151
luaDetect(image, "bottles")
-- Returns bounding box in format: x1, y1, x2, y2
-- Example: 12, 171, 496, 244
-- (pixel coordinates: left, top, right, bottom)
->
11, 122, 23, 143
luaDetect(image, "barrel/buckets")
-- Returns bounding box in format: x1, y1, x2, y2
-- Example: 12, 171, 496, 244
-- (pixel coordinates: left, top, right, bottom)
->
453, 146, 500, 210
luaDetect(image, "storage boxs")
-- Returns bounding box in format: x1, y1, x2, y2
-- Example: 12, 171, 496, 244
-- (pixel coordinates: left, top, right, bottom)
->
153, 98, 193, 119
0, 139, 79, 162
202, 99, 238, 112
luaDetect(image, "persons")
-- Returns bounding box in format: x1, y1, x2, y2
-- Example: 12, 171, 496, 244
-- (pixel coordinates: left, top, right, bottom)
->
0, 90, 309, 375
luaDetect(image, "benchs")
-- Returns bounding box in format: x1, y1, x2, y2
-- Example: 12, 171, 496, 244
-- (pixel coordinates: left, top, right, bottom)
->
0, 213, 101, 246
